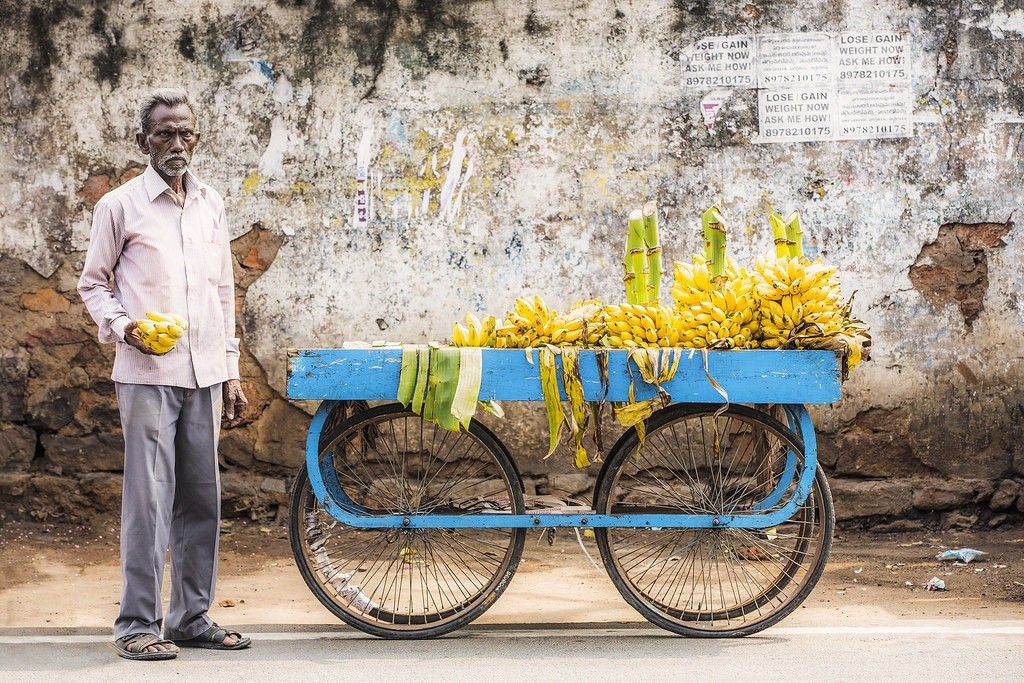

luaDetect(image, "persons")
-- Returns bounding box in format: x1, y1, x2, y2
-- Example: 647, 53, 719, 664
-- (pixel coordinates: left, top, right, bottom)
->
77, 90, 252, 659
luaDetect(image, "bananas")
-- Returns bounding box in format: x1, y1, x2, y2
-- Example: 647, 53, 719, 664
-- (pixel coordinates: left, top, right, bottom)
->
132, 311, 186, 354
452, 253, 848, 349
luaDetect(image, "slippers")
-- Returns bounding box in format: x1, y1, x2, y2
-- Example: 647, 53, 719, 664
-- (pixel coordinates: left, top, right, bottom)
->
173, 621, 252, 649
107, 631, 179, 660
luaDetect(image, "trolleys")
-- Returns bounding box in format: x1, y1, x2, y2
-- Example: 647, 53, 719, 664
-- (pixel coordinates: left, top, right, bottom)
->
287, 340, 843, 638
